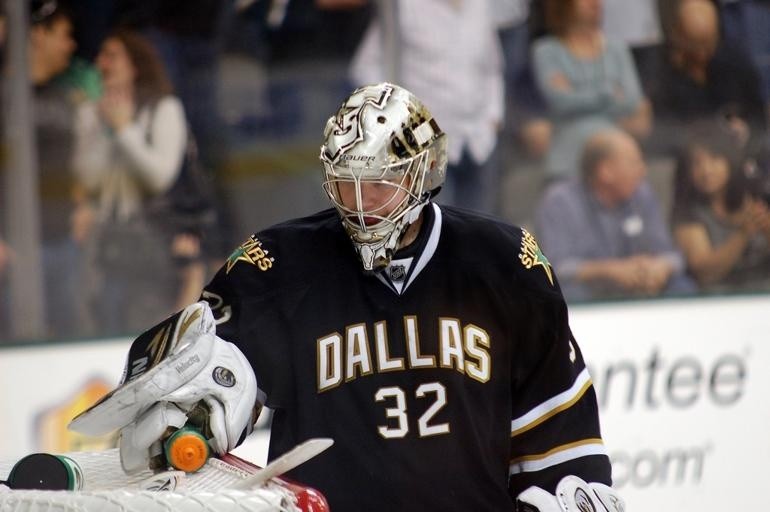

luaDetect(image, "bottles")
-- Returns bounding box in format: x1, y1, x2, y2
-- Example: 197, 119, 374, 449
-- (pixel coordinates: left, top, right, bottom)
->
163, 423, 212, 472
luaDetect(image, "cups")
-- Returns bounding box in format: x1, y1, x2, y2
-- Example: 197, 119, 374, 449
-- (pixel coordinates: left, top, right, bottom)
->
0, 454, 84, 491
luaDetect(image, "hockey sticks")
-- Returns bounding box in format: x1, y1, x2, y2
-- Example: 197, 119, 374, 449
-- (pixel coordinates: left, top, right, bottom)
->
233, 437, 334, 489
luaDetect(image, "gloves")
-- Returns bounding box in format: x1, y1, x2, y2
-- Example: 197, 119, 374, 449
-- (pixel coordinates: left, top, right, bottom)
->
147, 398, 218, 476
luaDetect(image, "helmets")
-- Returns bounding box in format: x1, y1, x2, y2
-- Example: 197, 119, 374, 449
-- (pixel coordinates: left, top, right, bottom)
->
318, 82, 449, 210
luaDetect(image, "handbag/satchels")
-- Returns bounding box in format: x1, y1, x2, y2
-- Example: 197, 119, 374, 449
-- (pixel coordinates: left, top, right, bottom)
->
142, 92, 215, 218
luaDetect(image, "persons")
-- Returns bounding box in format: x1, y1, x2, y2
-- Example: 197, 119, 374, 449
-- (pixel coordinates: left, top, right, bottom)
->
0, 0, 769, 333
67, 82, 627, 512
